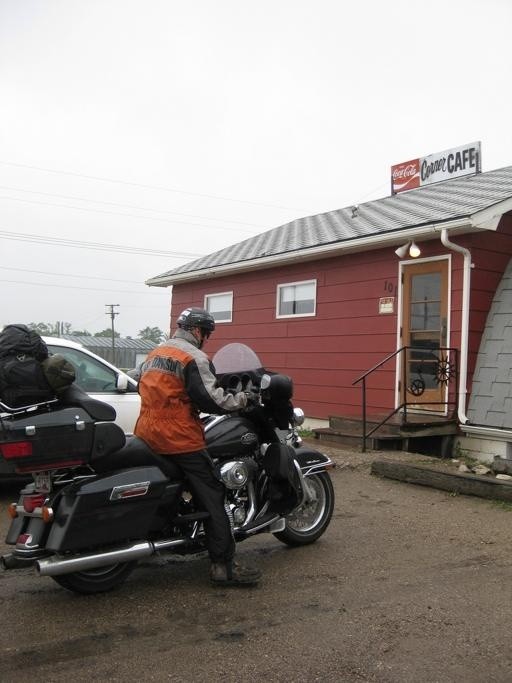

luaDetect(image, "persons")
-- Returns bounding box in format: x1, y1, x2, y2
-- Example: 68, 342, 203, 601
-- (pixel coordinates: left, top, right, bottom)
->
132, 305, 264, 583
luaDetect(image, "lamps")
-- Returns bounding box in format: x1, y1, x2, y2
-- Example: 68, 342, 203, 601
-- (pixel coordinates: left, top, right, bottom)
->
393, 238, 422, 258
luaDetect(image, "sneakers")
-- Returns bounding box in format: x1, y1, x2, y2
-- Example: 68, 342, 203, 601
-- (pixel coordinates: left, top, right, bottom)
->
210, 560, 263, 584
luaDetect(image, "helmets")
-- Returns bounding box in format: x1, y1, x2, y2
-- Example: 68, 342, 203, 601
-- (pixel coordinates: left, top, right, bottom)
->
176, 307, 215, 331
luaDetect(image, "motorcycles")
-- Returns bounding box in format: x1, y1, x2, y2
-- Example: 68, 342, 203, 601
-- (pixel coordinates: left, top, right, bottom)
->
1, 339, 338, 601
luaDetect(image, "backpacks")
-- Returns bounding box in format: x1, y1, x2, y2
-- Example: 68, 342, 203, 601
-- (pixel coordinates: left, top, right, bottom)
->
0, 323, 75, 413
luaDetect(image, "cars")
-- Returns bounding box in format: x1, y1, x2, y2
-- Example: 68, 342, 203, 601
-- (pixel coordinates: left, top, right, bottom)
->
408, 348, 440, 389
38, 336, 214, 443
